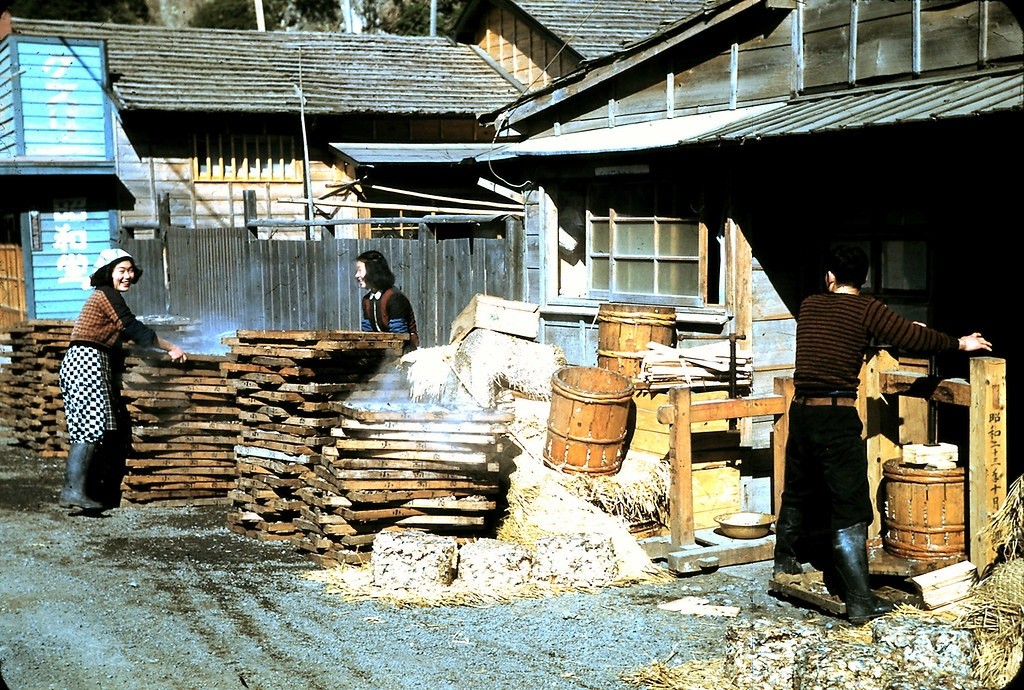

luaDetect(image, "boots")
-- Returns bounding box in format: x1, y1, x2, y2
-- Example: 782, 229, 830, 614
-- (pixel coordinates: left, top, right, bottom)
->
831, 523, 899, 623
60, 443, 103, 510
773, 506, 810, 574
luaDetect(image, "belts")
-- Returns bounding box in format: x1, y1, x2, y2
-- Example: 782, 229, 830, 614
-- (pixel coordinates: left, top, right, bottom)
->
792, 395, 855, 406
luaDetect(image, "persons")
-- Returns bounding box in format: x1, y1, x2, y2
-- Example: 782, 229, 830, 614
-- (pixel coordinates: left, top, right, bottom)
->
769, 244, 997, 624
58, 247, 185, 510
350, 248, 421, 344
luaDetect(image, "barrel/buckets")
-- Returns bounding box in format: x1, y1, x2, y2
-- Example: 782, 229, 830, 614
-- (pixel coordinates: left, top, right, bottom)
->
595, 303, 675, 380
542, 365, 635, 476
882, 457, 968, 561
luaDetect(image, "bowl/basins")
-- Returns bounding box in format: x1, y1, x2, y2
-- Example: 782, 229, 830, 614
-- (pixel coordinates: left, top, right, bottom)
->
713, 512, 777, 539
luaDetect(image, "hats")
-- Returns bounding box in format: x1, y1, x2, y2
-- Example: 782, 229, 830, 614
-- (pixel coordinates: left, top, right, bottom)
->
91, 248, 132, 276
